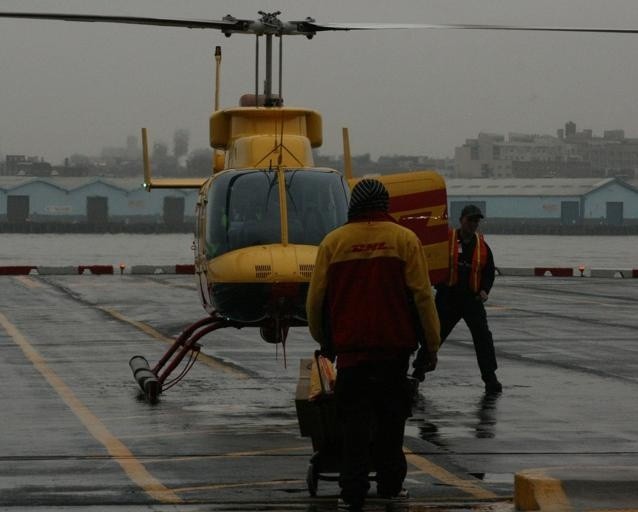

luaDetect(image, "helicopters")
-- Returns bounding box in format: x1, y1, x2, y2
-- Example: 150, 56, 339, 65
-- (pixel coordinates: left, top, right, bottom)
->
0, 11, 637, 401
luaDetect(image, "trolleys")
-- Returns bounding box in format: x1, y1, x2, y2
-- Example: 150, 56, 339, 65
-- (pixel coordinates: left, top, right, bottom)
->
306, 348, 410, 498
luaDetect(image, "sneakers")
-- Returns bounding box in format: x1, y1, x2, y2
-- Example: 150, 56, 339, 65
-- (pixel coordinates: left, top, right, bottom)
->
336, 498, 361, 508
485, 381, 502, 394
412, 369, 425, 382
377, 488, 409, 501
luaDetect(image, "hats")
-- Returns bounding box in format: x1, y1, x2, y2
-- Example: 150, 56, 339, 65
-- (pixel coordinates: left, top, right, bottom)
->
461, 205, 484, 219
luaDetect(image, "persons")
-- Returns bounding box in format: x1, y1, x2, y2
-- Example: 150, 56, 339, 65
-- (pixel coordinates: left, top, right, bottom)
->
304, 178, 443, 506
411, 203, 502, 394
409, 395, 500, 483
